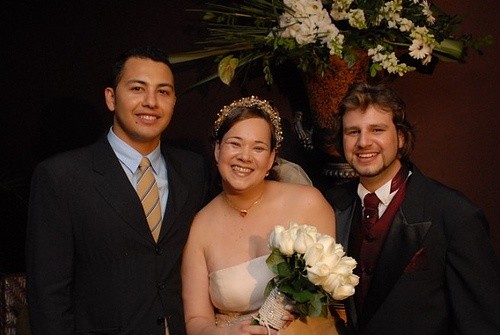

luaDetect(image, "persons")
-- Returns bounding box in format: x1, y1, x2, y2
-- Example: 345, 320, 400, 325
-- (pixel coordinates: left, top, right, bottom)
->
23, 45, 223, 335
178, 96, 337, 335
320, 81, 500, 335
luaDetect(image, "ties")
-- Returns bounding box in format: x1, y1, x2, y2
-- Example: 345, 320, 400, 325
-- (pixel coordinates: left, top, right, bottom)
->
362, 165, 409, 230
136, 158, 170, 335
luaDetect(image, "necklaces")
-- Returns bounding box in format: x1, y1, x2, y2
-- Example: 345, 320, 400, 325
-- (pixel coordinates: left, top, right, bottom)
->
220, 180, 266, 218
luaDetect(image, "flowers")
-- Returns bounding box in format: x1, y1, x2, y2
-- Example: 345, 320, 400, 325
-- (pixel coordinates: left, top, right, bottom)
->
264, 0, 486, 79
249, 221, 362, 333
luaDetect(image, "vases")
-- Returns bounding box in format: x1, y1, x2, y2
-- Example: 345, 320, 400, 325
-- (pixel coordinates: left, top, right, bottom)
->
303, 46, 378, 131
